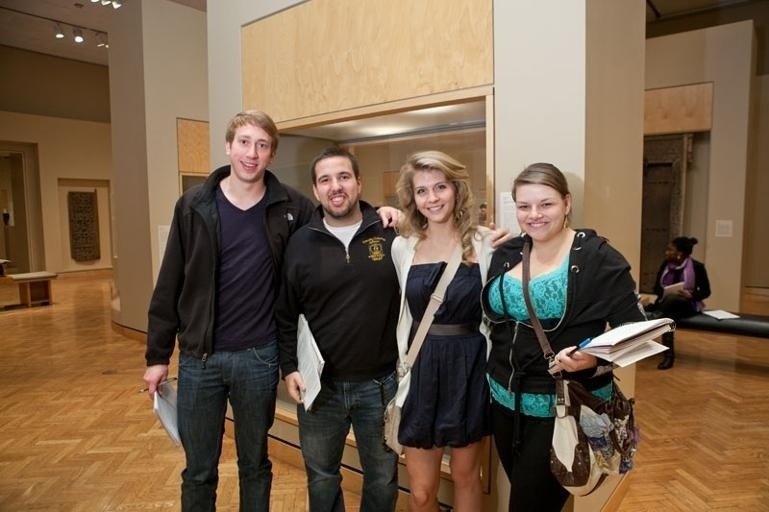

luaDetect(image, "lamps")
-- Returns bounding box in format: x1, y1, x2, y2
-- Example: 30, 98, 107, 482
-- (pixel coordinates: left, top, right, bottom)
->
53, 20, 107, 47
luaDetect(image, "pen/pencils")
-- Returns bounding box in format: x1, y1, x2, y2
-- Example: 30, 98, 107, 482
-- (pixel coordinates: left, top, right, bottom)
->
137, 377, 179, 395
558, 337, 593, 363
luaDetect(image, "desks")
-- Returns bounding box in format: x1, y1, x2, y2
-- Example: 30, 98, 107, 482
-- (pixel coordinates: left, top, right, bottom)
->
7, 270, 57, 309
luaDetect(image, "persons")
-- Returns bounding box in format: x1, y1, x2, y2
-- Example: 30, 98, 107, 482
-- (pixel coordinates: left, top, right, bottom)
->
478, 159, 648, 512
479, 202, 488, 227
381, 148, 504, 512
276, 144, 515, 512
142, 107, 412, 512
644, 234, 712, 372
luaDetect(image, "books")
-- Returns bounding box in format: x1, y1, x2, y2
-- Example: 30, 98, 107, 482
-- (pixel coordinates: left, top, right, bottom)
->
150, 378, 184, 450
294, 313, 326, 413
658, 280, 686, 304
703, 308, 742, 322
546, 315, 677, 381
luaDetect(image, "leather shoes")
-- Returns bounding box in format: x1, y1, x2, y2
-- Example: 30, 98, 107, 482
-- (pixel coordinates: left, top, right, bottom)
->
656, 355, 675, 369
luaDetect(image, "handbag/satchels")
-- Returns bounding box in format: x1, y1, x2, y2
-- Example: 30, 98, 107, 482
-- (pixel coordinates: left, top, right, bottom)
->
382, 368, 412, 457
548, 376, 637, 498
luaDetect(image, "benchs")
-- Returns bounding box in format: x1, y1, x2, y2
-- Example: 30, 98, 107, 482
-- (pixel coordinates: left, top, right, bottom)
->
646, 309, 769, 341
